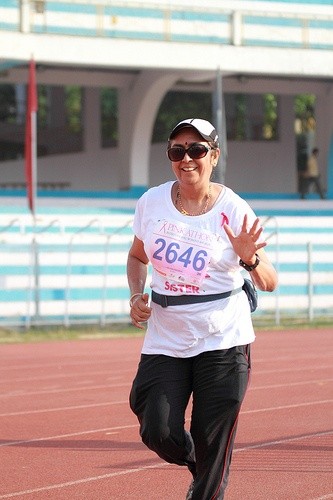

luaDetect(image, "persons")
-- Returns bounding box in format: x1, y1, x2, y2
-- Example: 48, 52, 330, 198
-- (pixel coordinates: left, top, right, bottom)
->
127, 119, 277, 500
301, 148, 324, 200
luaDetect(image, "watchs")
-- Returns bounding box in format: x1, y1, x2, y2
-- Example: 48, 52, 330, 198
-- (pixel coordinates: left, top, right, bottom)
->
239, 253, 261, 272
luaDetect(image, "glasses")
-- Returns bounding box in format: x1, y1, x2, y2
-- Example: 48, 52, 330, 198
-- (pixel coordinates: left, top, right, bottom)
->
168, 144, 214, 161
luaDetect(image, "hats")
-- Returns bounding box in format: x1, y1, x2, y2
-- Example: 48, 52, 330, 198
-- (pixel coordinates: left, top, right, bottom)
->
168, 119, 218, 148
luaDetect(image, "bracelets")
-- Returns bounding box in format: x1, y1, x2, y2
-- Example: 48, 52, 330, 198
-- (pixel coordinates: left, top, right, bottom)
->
129, 293, 149, 309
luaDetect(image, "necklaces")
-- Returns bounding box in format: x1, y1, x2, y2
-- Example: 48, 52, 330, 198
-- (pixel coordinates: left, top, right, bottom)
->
178, 180, 213, 216
